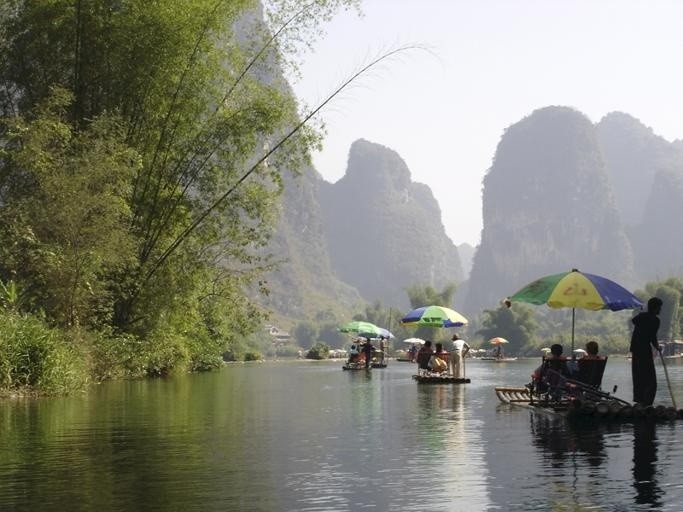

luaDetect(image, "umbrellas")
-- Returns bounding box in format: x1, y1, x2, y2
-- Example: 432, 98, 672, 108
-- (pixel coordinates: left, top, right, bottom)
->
486, 336, 508, 345
397, 303, 469, 341
401, 337, 427, 346
337, 320, 379, 338
499, 267, 645, 358
355, 326, 394, 340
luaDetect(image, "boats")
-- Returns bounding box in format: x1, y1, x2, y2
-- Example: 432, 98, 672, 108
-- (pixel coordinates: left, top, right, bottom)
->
342, 354, 519, 384
495, 385, 683, 423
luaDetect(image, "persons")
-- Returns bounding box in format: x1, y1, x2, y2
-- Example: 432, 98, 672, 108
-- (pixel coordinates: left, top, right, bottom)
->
369, 346, 380, 363
567, 340, 603, 402
431, 343, 448, 372
343, 344, 360, 365
409, 344, 417, 363
447, 333, 472, 377
378, 336, 389, 365
485, 344, 506, 359
359, 338, 376, 367
350, 337, 362, 353
629, 294, 663, 407
414, 340, 432, 371
523, 343, 567, 393
406, 344, 412, 361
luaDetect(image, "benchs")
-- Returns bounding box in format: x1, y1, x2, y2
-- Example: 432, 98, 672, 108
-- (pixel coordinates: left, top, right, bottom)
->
416, 350, 455, 377
523, 354, 609, 407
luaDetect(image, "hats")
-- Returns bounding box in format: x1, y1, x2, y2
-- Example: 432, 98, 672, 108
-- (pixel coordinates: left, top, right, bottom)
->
450, 333, 461, 340
